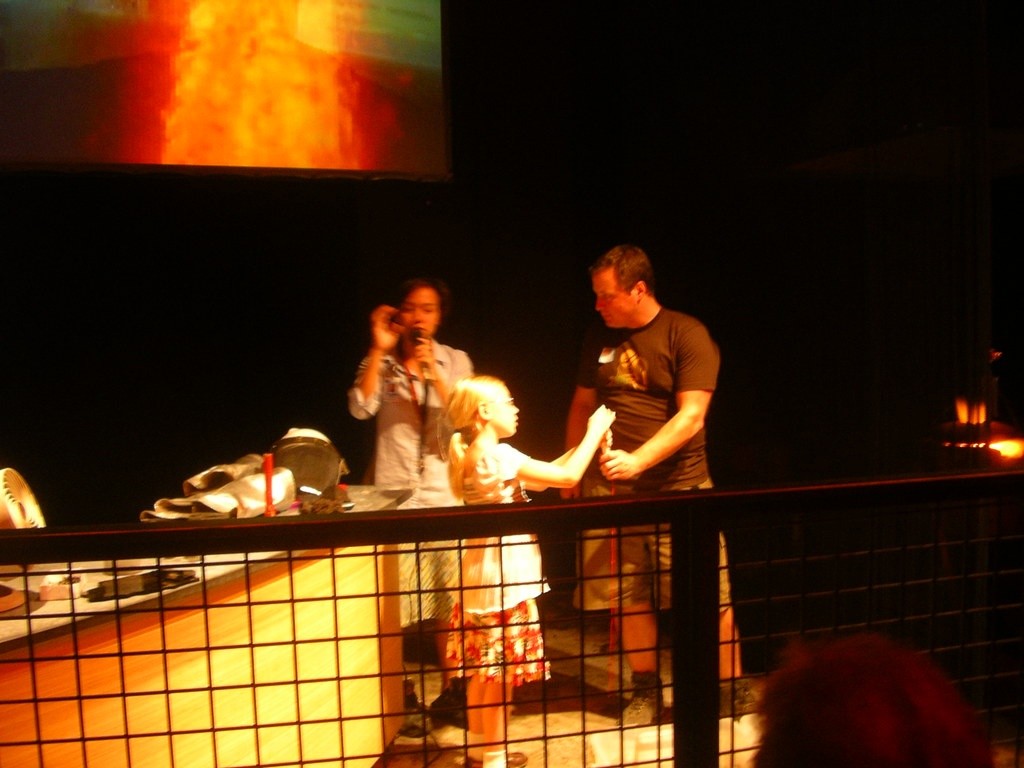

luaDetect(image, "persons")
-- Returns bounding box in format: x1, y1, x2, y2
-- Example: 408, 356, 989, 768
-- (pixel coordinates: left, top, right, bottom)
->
348, 278, 476, 738
446, 376, 617, 768
559, 245, 759, 728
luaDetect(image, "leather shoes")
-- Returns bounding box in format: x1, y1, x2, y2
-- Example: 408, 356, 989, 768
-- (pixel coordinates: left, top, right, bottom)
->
465, 752, 528, 768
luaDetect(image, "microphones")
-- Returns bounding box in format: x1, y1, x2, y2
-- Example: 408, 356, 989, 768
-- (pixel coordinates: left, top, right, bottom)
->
410, 329, 435, 384
600, 428, 613, 455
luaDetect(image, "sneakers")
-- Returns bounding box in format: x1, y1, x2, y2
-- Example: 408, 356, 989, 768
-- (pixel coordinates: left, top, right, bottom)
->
619, 673, 665, 727
719, 681, 760, 718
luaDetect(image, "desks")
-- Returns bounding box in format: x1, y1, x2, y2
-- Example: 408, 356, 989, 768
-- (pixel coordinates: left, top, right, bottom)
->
0, 485, 421, 768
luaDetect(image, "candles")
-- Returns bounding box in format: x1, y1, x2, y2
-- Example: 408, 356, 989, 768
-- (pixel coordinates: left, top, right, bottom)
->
262, 453, 274, 511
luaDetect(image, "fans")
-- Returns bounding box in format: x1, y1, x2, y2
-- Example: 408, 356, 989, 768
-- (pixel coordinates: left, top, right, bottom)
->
0, 467, 47, 614
